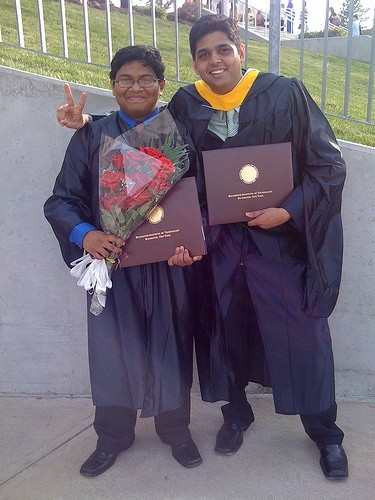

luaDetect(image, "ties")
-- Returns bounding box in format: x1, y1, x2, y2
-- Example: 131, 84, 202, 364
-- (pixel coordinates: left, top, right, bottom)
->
134, 123, 145, 150
226, 109, 239, 137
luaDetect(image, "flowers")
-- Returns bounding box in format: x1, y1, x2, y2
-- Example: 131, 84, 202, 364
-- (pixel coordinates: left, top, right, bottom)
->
70, 109, 190, 316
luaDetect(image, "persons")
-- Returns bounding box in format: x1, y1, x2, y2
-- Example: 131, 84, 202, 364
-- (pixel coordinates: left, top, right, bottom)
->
186, 0, 308, 35
41, 45, 223, 478
57, 13, 349, 481
329, 7, 339, 31
351, 14, 360, 37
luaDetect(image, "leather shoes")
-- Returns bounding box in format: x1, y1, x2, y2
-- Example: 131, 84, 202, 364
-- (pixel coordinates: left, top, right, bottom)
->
317, 442, 348, 480
215, 420, 249, 455
79, 438, 134, 476
157, 429, 202, 468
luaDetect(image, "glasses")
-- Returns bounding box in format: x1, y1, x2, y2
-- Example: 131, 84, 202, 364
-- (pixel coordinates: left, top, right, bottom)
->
114, 76, 160, 88
